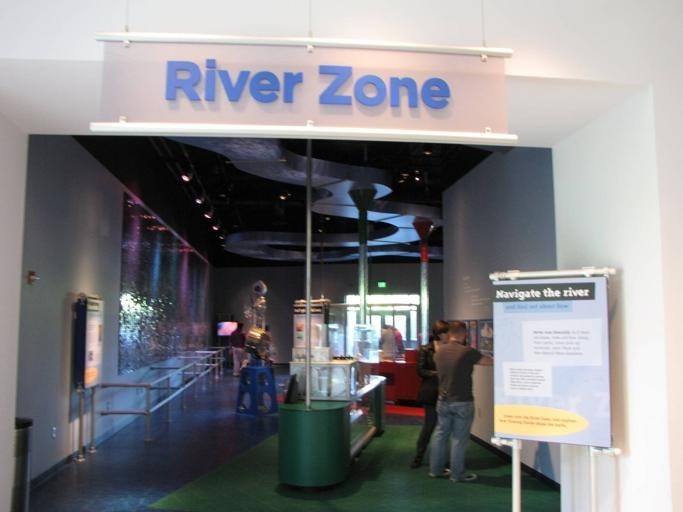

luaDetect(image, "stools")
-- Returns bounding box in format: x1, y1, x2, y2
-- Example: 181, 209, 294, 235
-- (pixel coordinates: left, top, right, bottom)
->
236, 365, 278, 416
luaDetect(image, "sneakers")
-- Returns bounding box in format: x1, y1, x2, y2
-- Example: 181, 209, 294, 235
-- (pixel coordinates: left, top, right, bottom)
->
452, 473, 477, 481
427, 469, 451, 478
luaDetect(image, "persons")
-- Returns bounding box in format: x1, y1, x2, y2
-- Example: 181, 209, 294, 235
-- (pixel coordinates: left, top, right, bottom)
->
230, 322, 247, 376
264, 324, 274, 364
410, 320, 450, 469
379, 324, 404, 358
428, 320, 494, 483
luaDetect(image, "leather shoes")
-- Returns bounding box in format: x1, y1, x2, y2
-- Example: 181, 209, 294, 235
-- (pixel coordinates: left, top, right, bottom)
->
410, 458, 421, 467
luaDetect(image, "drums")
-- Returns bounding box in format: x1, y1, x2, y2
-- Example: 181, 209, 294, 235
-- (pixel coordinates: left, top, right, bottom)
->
242, 296, 272, 359
